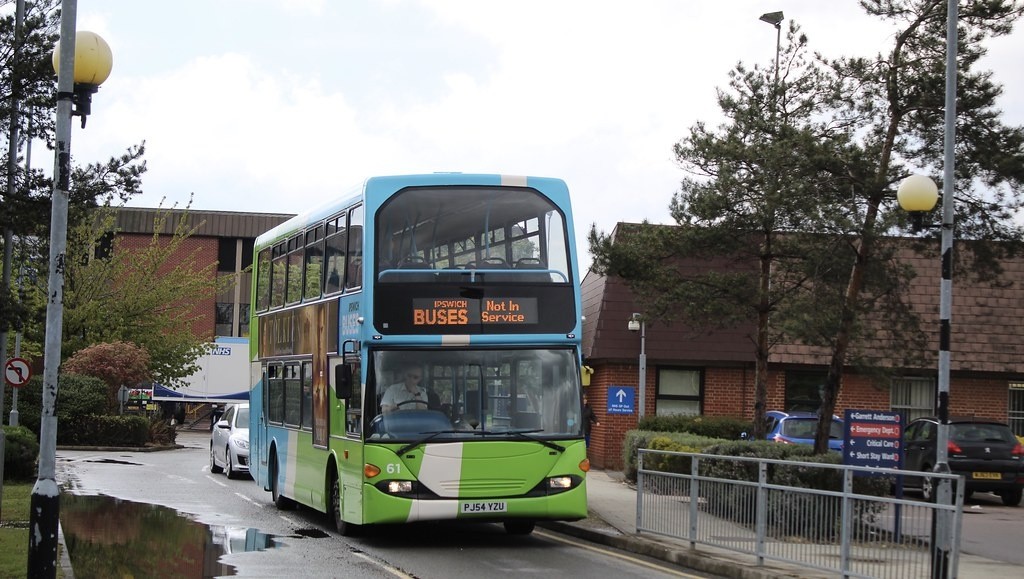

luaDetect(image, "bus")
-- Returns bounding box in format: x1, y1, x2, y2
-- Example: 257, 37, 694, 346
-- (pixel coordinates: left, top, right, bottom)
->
248, 170, 593, 537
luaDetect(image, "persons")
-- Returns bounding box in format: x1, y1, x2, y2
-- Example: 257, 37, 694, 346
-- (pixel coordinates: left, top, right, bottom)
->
381, 363, 428, 413
584, 393, 600, 448
303, 385, 314, 420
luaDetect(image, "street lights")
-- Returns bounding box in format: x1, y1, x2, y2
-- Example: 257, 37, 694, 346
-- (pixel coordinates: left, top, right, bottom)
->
896, 0, 961, 579
757, 8, 786, 143
22, 0, 113, 579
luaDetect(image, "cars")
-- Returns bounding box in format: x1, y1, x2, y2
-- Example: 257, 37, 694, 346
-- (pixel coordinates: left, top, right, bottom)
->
900, 413, 1024, 506
209, 403, 253, 479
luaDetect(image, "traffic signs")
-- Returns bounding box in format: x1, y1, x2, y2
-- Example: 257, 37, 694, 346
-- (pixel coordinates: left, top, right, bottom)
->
842, 408, 901, 475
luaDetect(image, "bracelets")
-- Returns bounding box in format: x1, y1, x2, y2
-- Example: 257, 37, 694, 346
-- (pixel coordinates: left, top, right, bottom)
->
415, 392, 422, 396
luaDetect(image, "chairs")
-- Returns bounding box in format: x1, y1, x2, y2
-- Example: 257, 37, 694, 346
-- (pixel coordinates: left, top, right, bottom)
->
356, 255, 553, 288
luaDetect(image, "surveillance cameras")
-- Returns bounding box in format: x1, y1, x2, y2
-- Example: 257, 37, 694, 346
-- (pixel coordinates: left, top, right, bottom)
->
629, 321, 640, 333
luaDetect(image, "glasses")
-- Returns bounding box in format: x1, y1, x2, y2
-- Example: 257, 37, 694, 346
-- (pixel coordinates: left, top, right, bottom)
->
408, 373, 419, 380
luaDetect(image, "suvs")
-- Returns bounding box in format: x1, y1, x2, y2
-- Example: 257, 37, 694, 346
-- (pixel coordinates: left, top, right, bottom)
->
740, 411, 846, 453
